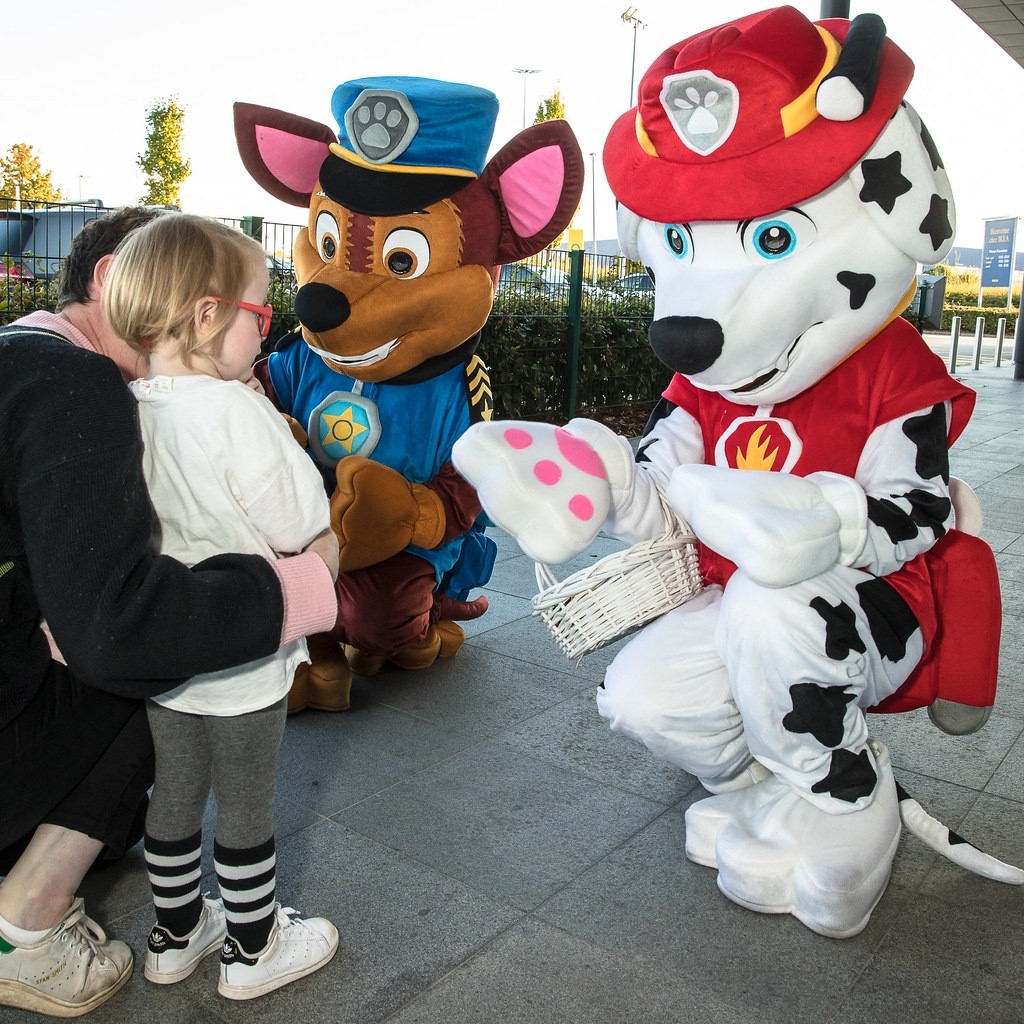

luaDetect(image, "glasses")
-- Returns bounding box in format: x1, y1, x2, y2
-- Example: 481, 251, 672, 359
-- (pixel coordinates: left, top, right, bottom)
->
206, 294, 273, 336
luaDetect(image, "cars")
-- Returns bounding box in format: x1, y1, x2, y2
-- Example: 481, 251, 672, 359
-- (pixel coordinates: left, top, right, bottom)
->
0, 209, 294, 304
607, 273, 657, 301
492, 262, 631, 309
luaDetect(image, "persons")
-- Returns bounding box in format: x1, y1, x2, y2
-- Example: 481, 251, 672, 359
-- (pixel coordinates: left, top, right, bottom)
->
101, 216, 342, 1000
0, 208, 340, 1024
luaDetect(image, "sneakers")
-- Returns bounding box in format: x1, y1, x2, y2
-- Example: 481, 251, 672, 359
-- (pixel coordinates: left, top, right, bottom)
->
216, 899, 339, 1000
145, 892, 228, 984
0, 897, 134, 1016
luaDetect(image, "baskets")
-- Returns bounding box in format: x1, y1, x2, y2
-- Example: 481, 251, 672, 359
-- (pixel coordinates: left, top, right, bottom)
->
530, 462, 701, 660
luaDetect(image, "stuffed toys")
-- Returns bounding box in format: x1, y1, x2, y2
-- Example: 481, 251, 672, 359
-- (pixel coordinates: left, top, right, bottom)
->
232, 74, 584, 713
453, 5, 1024, 937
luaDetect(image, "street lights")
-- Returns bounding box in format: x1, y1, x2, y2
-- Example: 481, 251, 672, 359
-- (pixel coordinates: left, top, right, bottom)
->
588, 152, 598, 286
510, 67, 542, 132
622, 5, 651, 111
